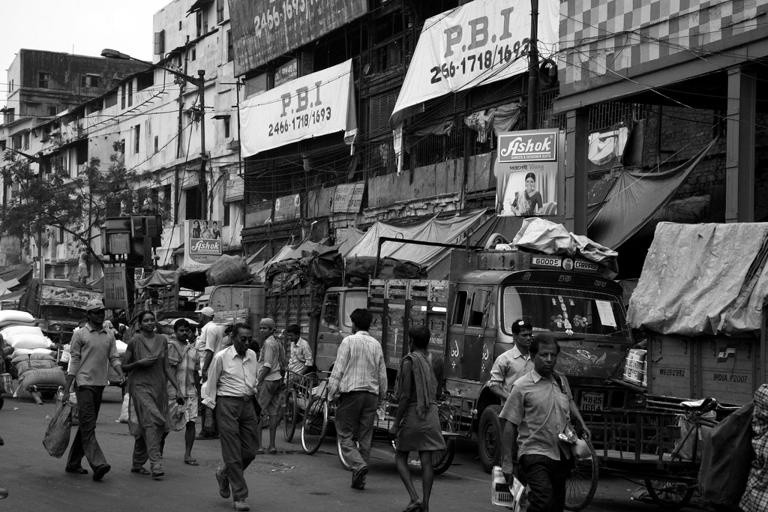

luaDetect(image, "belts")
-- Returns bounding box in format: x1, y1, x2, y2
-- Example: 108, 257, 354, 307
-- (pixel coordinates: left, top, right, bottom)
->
226, 396, 256, 401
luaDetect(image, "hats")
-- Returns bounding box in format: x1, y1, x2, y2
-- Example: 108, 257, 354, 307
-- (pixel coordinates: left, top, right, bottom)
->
195, 307, 215, 317
86, 299, 105, 311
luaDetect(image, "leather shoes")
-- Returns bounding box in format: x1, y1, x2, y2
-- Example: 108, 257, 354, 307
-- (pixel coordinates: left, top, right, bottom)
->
65, 467, 88, 474
93, 464, 110, 480
132, 467, 150, 475
152, 469, 164, 476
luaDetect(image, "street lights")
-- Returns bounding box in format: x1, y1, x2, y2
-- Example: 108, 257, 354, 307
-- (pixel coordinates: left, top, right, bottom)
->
98, 46, 207, 220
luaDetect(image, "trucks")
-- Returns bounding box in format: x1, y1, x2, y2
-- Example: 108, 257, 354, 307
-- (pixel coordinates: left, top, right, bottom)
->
269, 218, 669, 462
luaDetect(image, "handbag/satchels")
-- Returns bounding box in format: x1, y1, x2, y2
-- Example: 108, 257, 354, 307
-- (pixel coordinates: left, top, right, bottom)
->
42, 401, 72, 456
570, 438, 593, 465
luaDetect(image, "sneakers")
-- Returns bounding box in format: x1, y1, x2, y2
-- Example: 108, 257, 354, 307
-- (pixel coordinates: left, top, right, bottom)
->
351, 466, 368, 488
233, 500, 250, 510
404, 499, 421, 512
216, 470, 230, 498
185, 458, 198, 465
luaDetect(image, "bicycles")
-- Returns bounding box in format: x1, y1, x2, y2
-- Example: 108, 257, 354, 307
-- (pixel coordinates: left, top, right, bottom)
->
333, 396, 461, 471
275, 368, 339, 454
645, 396, 752, 498
556, 446, 602, 509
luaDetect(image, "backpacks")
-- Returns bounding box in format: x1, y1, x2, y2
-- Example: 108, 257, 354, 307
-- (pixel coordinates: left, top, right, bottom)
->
699, 400, 754, 503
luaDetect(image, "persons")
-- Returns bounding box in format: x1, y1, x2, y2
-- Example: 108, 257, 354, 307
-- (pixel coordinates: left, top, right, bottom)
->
61, 299, 127, 481
102, 307, 311, 478
501, 334, 593, 511
323, 308, 390, 490
392, 327, 447, 512
510, 171, 545, 214
486, 317, 539, 491
190, 222, 222, 238
202, 322, 264, 510
